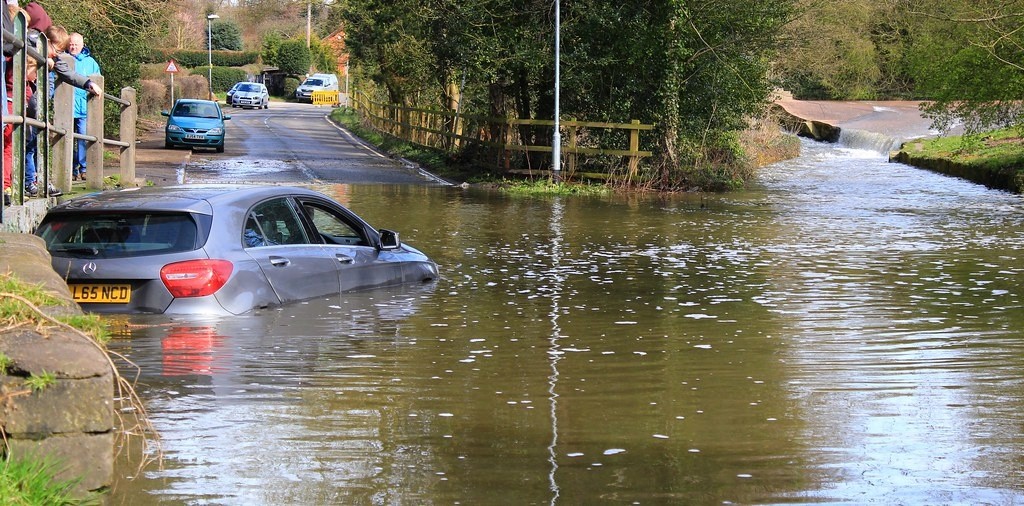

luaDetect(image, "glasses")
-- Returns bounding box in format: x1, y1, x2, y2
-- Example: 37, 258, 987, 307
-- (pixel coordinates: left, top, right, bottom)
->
51, 41, 64, 57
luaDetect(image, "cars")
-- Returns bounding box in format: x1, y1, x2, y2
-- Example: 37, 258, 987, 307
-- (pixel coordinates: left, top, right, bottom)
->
29, 184, 438, 320
160, 98, 232, 154
226, 81, 254, 106
232, 82, 270, 110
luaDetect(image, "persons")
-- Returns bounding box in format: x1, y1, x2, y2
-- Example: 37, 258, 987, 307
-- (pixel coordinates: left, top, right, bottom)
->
0, 0, 101, 206
243, 211, 277, 247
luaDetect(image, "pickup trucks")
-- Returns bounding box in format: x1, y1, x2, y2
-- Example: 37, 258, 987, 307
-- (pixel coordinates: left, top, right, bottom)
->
294, 73, 339, 102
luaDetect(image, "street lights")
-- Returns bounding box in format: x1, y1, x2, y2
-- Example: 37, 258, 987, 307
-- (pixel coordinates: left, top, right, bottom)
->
206, 14, 220, 104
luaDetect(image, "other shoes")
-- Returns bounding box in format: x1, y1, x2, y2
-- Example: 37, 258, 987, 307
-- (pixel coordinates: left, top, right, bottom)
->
24, 183, 38, 196
72, 175, 78, 180
4, 195, 14, 206
82, 172, 86, 180
44, 183, 63, 197
